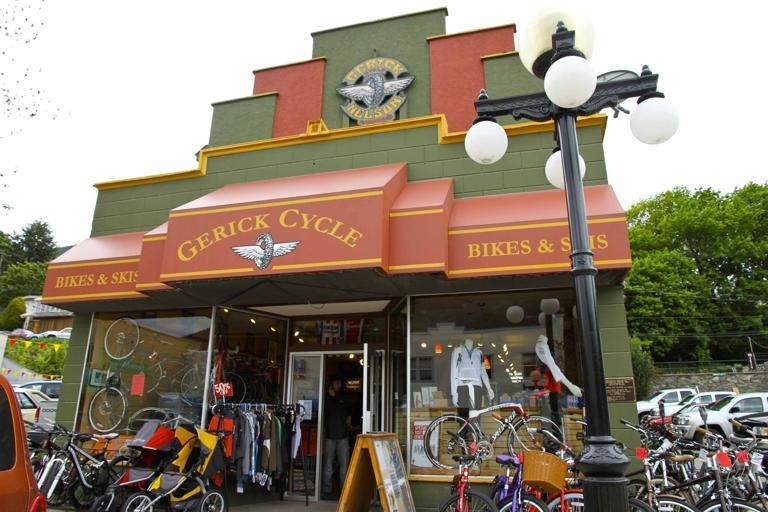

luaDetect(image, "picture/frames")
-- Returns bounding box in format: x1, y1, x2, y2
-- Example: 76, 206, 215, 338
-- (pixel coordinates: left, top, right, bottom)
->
89, 367, 114, 388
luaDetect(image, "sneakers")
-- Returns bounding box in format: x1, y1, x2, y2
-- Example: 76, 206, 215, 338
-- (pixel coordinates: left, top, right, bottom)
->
321, 492, 329, 499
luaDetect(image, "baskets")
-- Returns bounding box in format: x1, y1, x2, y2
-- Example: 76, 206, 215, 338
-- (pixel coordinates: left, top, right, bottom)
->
26, 431, 49, 450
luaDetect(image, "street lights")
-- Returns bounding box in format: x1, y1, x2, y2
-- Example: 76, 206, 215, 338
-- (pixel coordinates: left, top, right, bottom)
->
504, 298, 580, 442
464, 6, 676, 509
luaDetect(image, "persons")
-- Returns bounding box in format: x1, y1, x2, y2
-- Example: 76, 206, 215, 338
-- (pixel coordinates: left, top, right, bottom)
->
533, 332, 582, 453
323, 373, 354, 499
448, 337, 495, 461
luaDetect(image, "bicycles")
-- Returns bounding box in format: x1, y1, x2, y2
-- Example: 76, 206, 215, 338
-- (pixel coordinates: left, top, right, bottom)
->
23, 419, 134, 508
438, 401, 768, 512
87, 317, 272, 435
422, 399, 565, 470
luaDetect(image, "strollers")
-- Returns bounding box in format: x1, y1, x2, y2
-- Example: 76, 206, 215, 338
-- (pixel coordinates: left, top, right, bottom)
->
90, 420, 227, 512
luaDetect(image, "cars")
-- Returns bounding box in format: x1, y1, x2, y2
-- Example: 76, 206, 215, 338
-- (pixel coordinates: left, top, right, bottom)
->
9, 380, 60, 432
636, 387, 768, 452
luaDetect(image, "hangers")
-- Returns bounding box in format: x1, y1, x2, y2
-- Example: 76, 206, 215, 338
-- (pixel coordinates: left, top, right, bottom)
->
213, 402, 298, 422
